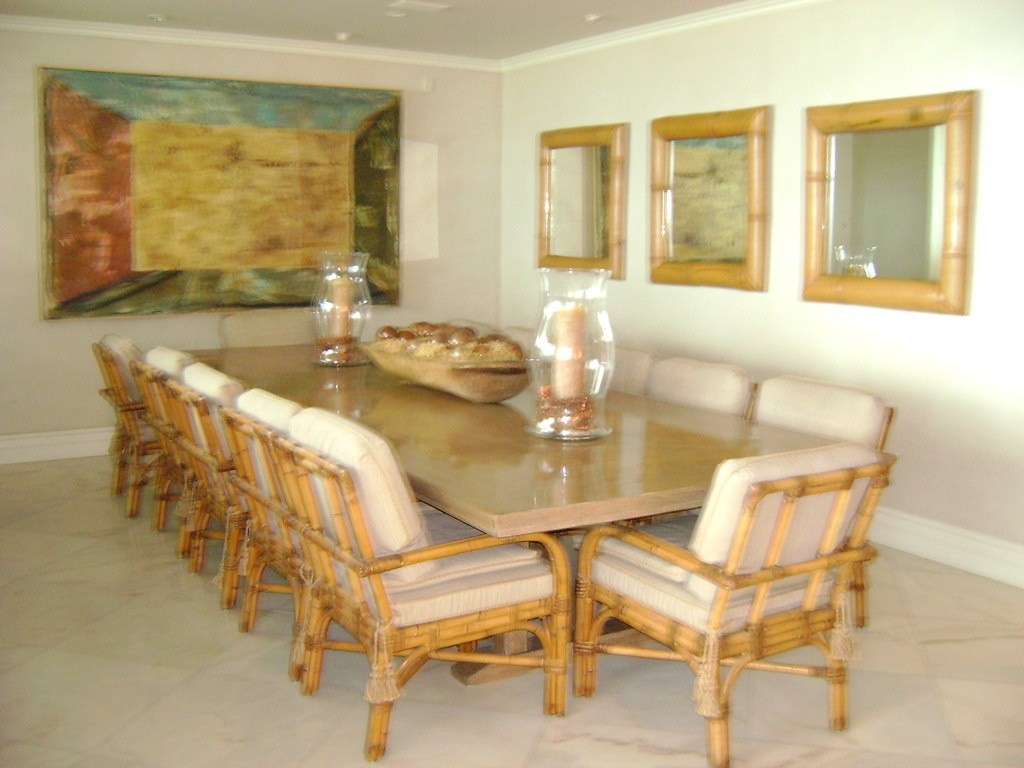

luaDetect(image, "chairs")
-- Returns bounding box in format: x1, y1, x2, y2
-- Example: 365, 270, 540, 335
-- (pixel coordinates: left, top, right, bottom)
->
92, 318, 899, 767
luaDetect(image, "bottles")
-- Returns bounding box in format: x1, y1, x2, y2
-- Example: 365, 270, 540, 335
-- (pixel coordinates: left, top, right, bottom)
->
835, 245, 877, 278
527, 268, 614, 439
314, 251, 374, 364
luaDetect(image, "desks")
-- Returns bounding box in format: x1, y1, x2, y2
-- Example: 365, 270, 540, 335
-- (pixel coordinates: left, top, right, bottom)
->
185, 340, 899, 687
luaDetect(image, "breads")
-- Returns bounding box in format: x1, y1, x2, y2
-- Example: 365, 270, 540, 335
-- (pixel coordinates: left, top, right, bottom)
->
374, 317, 523, 363
